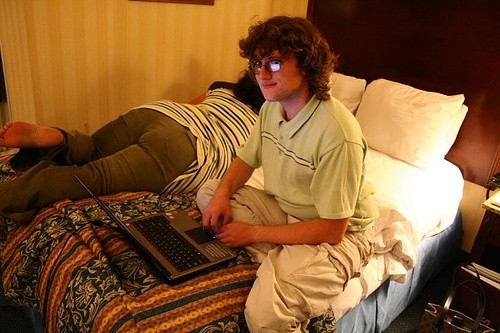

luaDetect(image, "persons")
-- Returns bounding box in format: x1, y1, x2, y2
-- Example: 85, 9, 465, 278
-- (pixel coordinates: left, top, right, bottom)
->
195, 15, 380, 333
0, 70, 266, 213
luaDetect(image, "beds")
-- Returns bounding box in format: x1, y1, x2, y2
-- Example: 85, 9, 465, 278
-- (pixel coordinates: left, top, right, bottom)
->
0, 0, 500, 333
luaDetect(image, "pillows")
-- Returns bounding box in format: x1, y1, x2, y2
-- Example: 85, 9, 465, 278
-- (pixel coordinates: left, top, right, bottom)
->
327, 72, 366, 114
355, 78, 469, 172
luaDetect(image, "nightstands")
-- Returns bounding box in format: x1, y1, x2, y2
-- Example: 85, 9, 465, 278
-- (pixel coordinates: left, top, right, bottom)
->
466, 193, 500, 283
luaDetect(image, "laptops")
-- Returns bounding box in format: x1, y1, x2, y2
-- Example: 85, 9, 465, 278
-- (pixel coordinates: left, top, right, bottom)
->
75, 176, 236, 283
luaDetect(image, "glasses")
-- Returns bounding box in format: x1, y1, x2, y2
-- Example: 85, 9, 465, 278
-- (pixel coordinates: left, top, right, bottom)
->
248, 58, 295, 75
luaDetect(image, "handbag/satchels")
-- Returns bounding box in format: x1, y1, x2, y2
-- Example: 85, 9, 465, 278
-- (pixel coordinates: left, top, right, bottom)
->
416, 263, 497, 333
